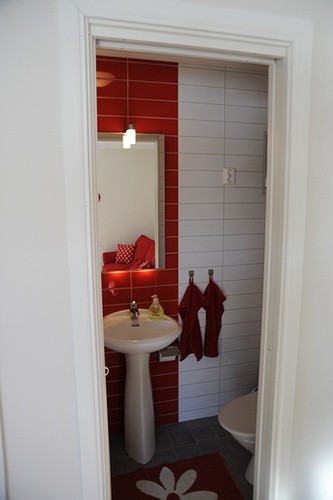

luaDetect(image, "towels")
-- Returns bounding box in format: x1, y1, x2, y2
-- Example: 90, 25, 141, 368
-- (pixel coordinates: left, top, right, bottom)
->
202, 275, 228, 359
177, 275, 212, 363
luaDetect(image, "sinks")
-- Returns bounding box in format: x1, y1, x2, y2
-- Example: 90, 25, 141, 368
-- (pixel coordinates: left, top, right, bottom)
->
104, 319, 178, 342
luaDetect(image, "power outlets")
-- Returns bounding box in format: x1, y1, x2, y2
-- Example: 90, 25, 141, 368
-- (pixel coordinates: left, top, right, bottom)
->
223, 167, 236, 184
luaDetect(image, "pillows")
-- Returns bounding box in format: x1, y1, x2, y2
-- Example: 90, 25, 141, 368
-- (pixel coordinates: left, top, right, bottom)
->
115, 243, 135, 264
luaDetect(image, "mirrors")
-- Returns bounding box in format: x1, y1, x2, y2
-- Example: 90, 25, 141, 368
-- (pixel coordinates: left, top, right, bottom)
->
100, 133, 164, 269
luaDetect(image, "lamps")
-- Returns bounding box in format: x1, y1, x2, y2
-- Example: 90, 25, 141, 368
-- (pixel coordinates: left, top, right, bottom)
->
125, 124, 139, 145
121, 137, 133, 150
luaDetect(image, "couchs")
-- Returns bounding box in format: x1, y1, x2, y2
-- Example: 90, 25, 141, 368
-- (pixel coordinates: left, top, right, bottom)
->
102, 234, 156, 269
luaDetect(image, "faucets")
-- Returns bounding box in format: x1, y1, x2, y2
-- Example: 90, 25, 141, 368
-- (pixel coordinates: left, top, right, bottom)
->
129, 300, 140, 321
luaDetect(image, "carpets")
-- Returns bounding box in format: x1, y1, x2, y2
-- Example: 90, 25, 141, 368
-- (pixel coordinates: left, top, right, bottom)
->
110, 452, 242, 500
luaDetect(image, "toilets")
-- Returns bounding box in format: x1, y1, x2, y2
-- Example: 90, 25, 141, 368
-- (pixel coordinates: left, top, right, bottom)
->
218, 390, 258, 486
